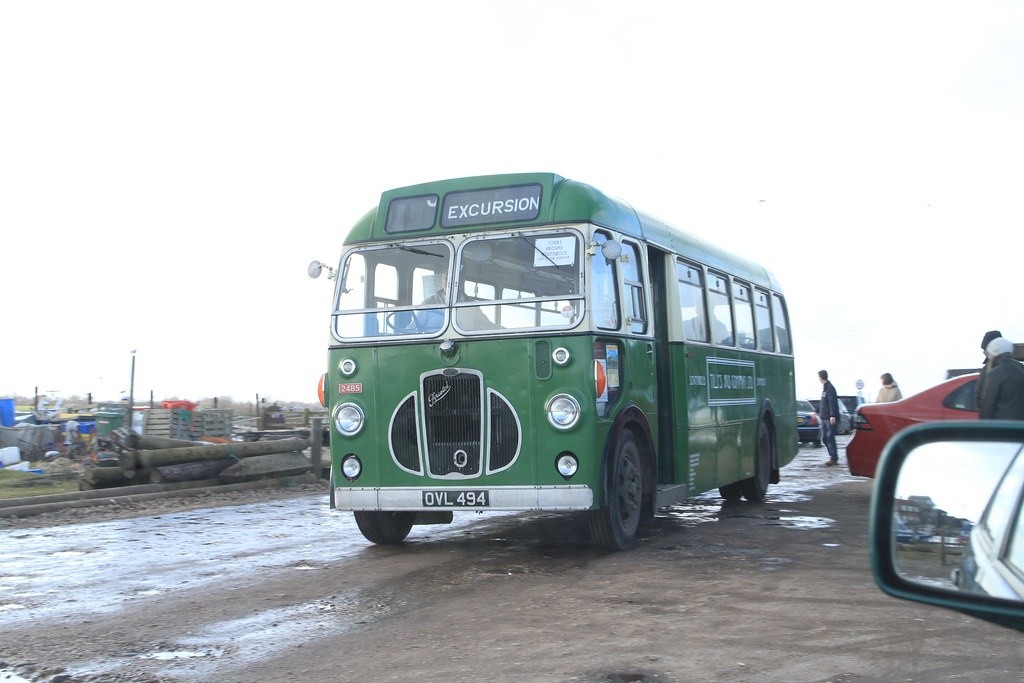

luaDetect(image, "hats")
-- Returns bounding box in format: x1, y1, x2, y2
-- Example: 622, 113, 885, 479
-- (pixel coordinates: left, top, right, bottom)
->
982, 330, 1002, 349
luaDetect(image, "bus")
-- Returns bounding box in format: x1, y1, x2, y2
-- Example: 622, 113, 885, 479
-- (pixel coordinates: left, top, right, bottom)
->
317, 172, 801, 551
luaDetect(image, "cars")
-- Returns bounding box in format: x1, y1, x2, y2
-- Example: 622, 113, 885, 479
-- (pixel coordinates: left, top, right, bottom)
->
797, 399, 822, 449
845, 372, 980, 480
808, 395, 867, 437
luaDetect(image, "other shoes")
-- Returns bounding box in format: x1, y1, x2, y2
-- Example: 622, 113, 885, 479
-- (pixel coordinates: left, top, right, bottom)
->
826, 457, 837, 466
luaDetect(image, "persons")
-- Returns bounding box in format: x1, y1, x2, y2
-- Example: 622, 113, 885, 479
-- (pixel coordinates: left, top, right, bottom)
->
682, 296, 729, 343
405, 265, 494, 330
818, 370, 839, 466
977, 330, 1024, 420
876, 373, 902, 403
